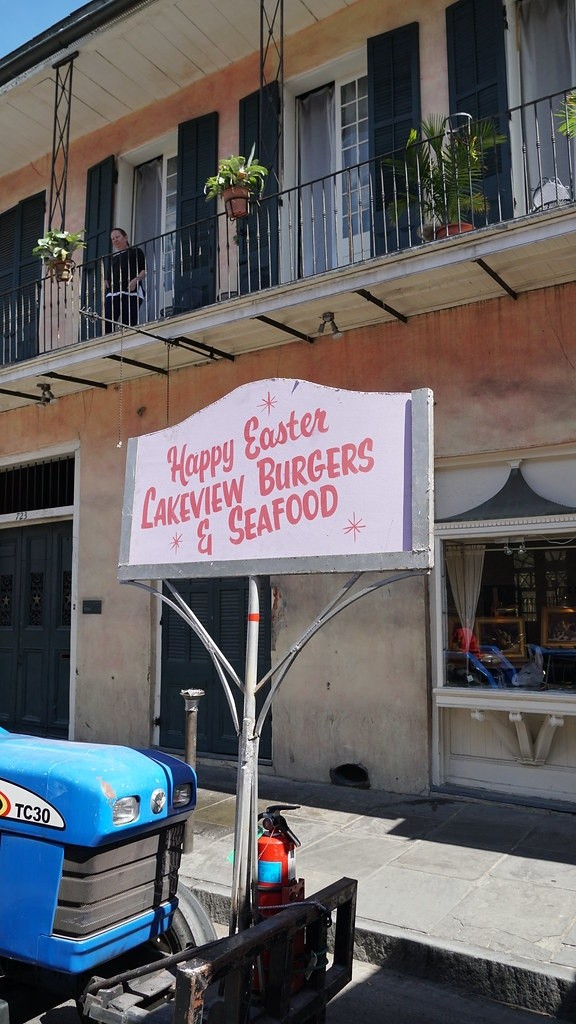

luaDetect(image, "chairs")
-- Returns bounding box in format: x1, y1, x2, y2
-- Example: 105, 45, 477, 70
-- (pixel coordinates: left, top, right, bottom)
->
528, 175, 573, 215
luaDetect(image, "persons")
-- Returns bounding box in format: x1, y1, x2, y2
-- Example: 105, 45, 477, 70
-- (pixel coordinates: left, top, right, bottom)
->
105, 228, 145, 334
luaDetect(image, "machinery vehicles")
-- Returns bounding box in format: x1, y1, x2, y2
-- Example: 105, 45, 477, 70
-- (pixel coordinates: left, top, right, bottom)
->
0, 724, 359, 1024
444, 643, 576, 692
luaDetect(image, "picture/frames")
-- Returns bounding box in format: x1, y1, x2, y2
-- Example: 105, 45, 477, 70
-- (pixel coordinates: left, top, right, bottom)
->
448, 616, 477, 651
475, 617, 526, 658
541, 606, 576, 648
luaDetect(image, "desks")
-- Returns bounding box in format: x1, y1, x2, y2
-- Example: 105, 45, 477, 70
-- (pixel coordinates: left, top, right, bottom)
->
532, 645, 576, 690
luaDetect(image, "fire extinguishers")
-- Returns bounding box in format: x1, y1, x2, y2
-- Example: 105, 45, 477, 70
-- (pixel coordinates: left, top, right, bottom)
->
252, 804, 302, 994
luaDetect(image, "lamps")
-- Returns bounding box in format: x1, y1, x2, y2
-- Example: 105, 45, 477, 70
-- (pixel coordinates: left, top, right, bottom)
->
504, 545, 513, 556
37, 383, 58, 407
316, 313, 343, 339
519, 545, 527, 555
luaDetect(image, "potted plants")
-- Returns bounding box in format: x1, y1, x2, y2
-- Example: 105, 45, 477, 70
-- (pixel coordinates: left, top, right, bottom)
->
380, 112, 508, 239
32, 228, 87, 282
205, 154, 268, 217
